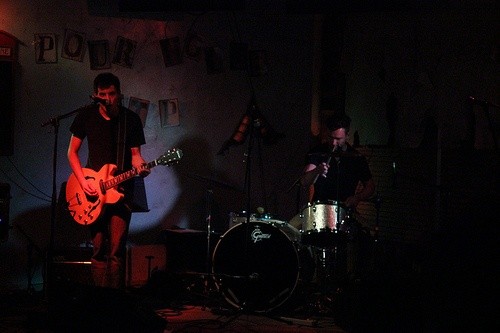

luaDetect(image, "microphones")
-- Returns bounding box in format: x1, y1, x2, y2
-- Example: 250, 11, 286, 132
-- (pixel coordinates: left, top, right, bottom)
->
247, 272, 261, 280
91, 95, 110, 106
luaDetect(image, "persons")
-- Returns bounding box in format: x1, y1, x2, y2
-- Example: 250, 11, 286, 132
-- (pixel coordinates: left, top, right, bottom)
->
290, 114, 373, 236
67, 73, 151, 280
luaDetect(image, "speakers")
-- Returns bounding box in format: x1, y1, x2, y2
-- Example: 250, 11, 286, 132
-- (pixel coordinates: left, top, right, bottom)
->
120, 244, 167, 287
52, 283, 168, 333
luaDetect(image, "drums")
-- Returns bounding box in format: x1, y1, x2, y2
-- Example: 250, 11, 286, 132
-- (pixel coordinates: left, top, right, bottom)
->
211, 220, 313, 312
300, 199, 350, 248
224, 209, 271, 229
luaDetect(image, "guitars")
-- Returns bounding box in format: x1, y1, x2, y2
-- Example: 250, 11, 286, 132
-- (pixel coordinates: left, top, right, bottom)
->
65, 146, 183, 227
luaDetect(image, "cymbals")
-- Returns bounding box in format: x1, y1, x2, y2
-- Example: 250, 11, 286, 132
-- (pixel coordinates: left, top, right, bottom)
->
182, 172, 237, 191
355, 144, 415, 155
308, 148, 369, 156
